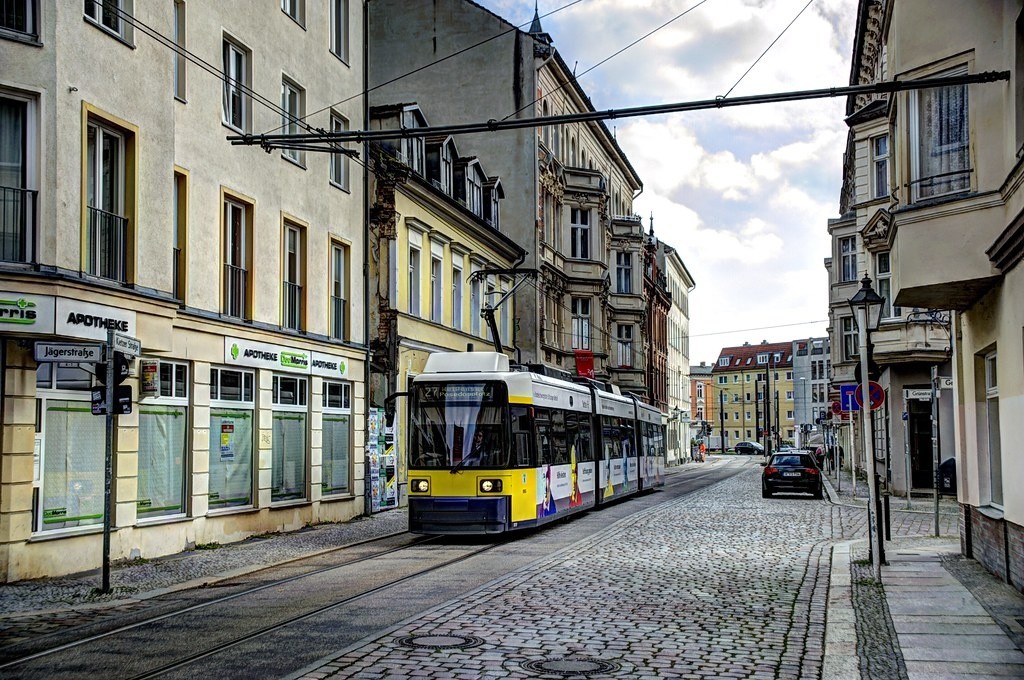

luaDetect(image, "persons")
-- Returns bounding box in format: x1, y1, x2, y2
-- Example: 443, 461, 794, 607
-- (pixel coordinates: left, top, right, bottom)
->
699, 442, 706, 462
468, 430, 490, 465
830, 443, 844, 470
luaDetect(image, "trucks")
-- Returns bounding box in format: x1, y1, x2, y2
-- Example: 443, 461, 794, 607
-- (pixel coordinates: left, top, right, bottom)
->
699, 436, 729, 451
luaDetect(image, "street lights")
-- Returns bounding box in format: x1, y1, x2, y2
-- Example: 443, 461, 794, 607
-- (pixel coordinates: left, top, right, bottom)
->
847, 268, 886, 566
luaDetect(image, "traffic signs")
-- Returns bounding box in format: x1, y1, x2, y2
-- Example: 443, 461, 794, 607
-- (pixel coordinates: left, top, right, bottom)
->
114, 332, 142, 357
33, 342, 103, 363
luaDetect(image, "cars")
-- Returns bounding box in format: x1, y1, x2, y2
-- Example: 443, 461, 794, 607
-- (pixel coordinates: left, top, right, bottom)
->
734, 441, 765, 455
778, 441, 844, 471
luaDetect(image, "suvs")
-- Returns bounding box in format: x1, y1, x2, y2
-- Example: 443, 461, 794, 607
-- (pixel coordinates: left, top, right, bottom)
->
761, 450, 823, 498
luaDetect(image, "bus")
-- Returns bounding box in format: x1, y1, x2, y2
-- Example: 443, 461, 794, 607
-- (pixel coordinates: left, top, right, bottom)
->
384, 268, 665, 535
